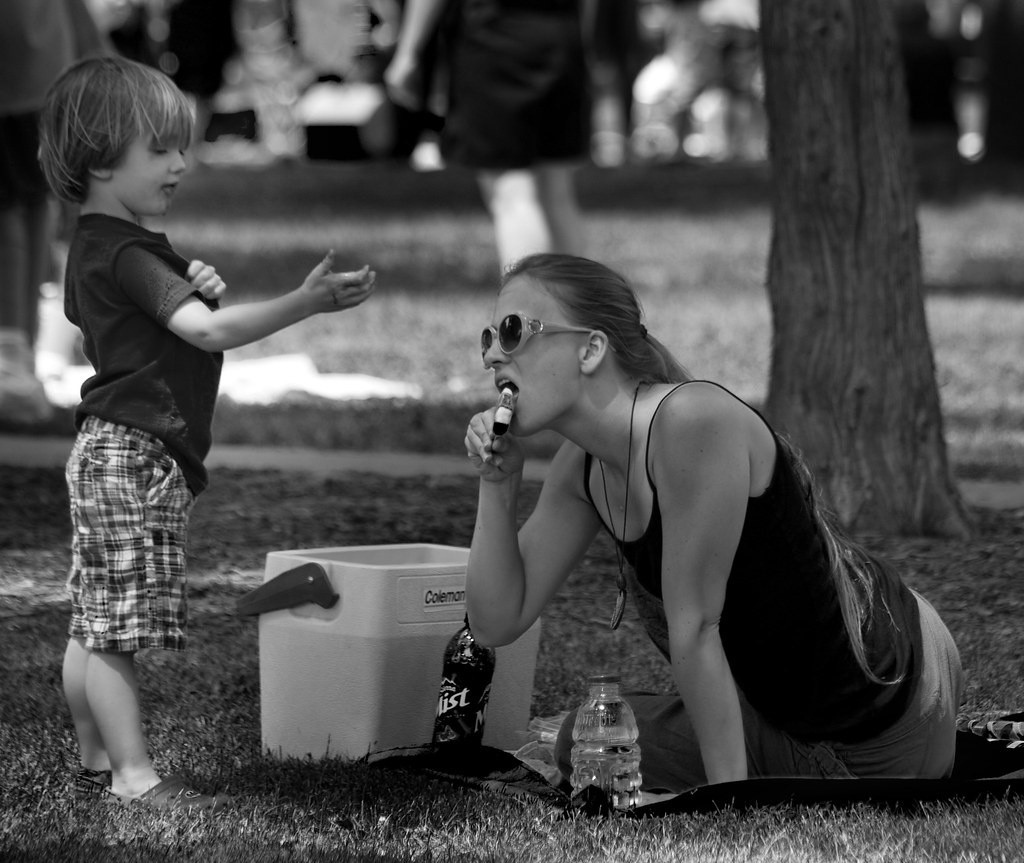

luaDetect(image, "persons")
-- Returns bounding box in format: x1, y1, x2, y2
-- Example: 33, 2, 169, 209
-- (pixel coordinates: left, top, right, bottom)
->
28, 56, 378, 813
383, 0, 599, 282
0, 0, 105, 346
465, 254, 962, 792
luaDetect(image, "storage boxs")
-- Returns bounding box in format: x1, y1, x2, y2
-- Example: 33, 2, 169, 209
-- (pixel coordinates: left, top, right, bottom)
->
255, 542, 544, 761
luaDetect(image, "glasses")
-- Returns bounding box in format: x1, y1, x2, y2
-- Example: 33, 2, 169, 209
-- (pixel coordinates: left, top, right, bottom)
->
480, 312, 617, 369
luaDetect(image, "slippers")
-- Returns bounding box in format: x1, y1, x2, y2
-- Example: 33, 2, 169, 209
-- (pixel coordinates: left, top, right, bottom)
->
75, 765, 228, 818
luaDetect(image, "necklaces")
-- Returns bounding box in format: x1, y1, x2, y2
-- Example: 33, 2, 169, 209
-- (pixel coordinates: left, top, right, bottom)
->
598, 386, 638, 631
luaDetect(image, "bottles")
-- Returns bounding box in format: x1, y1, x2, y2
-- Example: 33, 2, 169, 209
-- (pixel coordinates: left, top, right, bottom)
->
430, 612, 496, 752
572, 674, 641, 808
